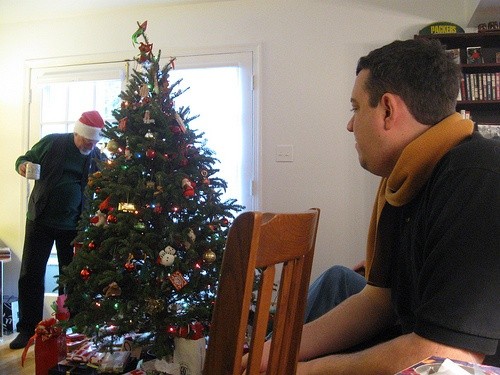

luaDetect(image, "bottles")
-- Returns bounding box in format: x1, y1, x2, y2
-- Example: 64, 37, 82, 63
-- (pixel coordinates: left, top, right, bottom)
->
460, 108, 471, 119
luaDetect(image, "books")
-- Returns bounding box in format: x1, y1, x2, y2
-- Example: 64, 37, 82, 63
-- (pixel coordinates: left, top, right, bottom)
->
460, 110, 470, 119
460, 72, 500, 100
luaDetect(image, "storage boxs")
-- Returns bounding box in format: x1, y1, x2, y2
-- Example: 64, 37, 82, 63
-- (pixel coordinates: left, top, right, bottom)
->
3, 296, 20, 335
34, 332, 152, 375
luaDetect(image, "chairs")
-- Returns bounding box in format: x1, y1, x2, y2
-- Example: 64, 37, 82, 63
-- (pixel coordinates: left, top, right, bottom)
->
200, 207, 321, 375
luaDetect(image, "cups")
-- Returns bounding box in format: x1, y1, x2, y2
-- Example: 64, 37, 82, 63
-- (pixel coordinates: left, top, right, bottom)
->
26, 163, 41, 180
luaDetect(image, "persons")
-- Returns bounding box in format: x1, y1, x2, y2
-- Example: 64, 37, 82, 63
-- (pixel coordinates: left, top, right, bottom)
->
10, 111, 110, 350
240, 38, 500, 375
265, 260, 368, 341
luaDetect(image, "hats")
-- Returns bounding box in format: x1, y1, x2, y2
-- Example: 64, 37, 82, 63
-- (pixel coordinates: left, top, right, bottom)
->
74, 111, 106, 140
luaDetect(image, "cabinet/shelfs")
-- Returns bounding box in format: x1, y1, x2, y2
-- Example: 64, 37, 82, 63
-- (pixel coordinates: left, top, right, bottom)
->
415, 31, 500, 139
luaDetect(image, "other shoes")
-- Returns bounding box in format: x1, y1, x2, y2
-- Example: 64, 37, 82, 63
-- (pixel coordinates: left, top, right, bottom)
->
10, 333, 33, 350
